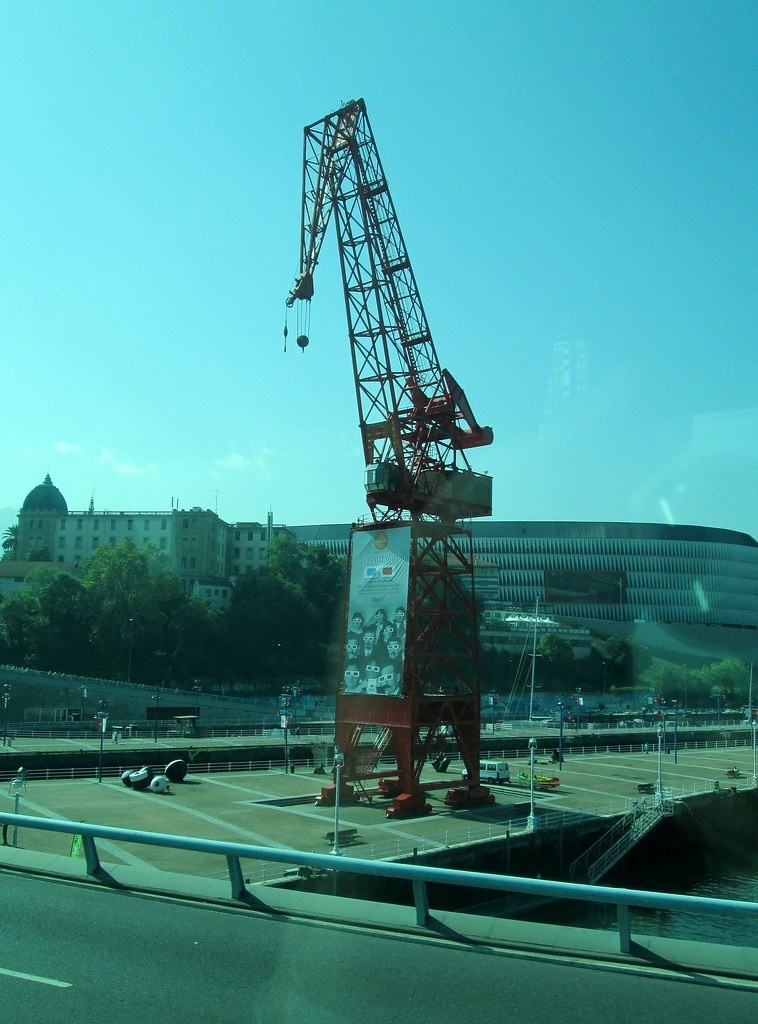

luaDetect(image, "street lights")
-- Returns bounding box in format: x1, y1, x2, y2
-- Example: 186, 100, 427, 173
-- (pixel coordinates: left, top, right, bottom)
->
671, 700, 677, 764
9, 768, 26, 846
660, 698, 666, 754
752, 720, 757, 778
657, 727, 663, 800
193, 680, 202, 716
278, 694, 290, 774
711, 692, 726, 725
277, 642, 281, 695
744, 660, 752, 725
329, 746, 344, 856
489, 689, 496, 734
96, 699, 109, 783
527, 736, 541, 830
1, 685, 12, 746
602, 661, 607, 694
127, 618, 135, 683
557, 699, 563, 772
575, 688, 582, 729
79, 685, 86, 720
152, 686, 162, 742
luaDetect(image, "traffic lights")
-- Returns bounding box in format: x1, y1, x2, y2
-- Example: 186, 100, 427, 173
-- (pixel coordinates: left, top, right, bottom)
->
571, 694, 578, 704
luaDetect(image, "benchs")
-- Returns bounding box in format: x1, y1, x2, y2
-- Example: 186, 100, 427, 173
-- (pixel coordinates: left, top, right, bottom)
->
633, 783, 657, 794
514, 801, 536, 814
322, 829, 363, 847
723, 770, 744, 779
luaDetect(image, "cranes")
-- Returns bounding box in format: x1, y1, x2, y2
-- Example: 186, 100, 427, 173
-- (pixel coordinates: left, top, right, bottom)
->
284, 97, 497, 817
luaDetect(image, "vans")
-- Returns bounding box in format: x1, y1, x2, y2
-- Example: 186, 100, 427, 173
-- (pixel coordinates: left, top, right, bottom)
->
462, 761, 510, 785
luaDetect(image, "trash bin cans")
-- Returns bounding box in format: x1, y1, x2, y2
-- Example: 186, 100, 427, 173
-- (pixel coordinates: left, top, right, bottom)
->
172, 715, 199, 732
112, 726, 133, 739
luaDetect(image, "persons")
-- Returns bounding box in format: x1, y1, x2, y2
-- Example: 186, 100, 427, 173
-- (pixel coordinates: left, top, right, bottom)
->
111, 730, 120, 744
734, 766, 739, 772
344, 606, 405, 695
294, 727, 301, 739
644, 742, 650, 754
